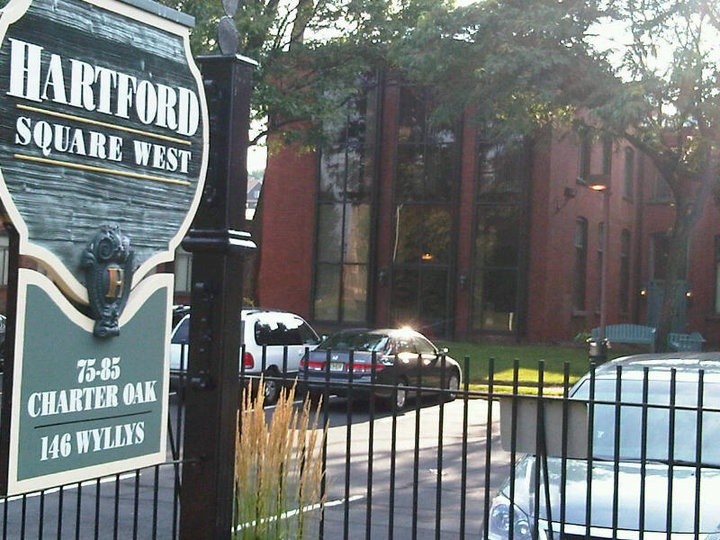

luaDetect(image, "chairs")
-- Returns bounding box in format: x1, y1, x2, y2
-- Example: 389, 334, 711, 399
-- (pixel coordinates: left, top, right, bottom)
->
665, 332, 707, 353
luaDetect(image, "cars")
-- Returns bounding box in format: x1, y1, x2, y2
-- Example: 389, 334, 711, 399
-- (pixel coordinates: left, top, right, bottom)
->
299, 326, 461, 410
484, 352, 720, 540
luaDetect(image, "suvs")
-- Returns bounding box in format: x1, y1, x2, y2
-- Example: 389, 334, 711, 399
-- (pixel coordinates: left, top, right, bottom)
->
170, 310, 321, 405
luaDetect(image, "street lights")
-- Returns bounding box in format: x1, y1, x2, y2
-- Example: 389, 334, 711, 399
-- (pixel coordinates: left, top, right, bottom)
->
586, 172, 611, 365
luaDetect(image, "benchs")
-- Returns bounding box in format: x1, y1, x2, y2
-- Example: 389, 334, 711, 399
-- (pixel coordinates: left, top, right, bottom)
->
590, 323, 658, 354
